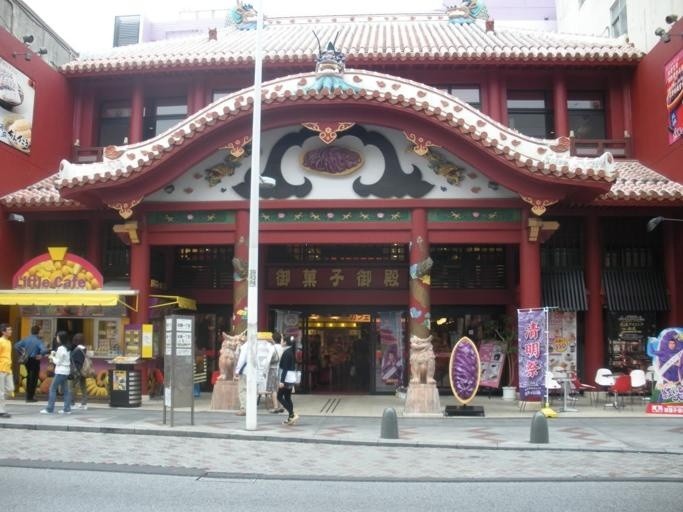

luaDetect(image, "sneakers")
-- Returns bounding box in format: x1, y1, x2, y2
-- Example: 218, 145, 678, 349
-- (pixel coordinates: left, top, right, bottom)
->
281, 414, 299, 425
0, 413, 12, 418
70, 404, 77, 409
39, 408, 53, 415
235, 409, 246, 416
76, 405, 87, 409
57, 410, 72, 414
26, 398, 38, 402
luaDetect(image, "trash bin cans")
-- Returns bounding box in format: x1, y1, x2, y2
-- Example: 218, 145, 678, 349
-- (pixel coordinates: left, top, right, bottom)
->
104, 353, 143, 409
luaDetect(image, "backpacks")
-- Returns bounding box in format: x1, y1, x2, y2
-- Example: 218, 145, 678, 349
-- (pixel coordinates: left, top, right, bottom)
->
77, 347, 96, 378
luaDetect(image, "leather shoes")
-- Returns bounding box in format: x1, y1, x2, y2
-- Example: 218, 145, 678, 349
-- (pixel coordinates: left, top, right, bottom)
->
269, 407, 284, 413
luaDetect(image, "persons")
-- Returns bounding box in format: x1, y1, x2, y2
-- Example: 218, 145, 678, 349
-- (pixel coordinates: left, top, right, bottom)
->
14, 325, 47, 402
69, 333, 88, 410
40, 330, 72, 416
649, 338, 680, 404
235, 330, 321, 425
0, 323, 15, 417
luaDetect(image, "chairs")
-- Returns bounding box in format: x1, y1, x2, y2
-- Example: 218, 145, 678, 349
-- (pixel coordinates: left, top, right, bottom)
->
545, 363, 660, 414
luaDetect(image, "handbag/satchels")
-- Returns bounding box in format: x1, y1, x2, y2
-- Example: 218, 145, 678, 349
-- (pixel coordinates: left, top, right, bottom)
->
239, 362, 247, 374
68, 365, 79, 380
278, 368, 301, 384
269, 351, 279, 364
18, 355, 28, 364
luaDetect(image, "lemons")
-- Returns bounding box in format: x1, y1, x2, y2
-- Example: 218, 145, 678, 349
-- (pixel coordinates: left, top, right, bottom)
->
17, 260, 99, 293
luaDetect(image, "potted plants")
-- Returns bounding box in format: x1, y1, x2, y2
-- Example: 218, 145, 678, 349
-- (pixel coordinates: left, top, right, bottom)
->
484, 311, 521, 400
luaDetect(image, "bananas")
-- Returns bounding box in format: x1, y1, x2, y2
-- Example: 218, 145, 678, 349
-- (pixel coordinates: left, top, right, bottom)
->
74, 377, 107, 399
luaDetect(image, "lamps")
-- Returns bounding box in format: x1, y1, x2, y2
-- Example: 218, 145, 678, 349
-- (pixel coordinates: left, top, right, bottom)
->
653, 12, 682, 45
11, 31, 48, 63
